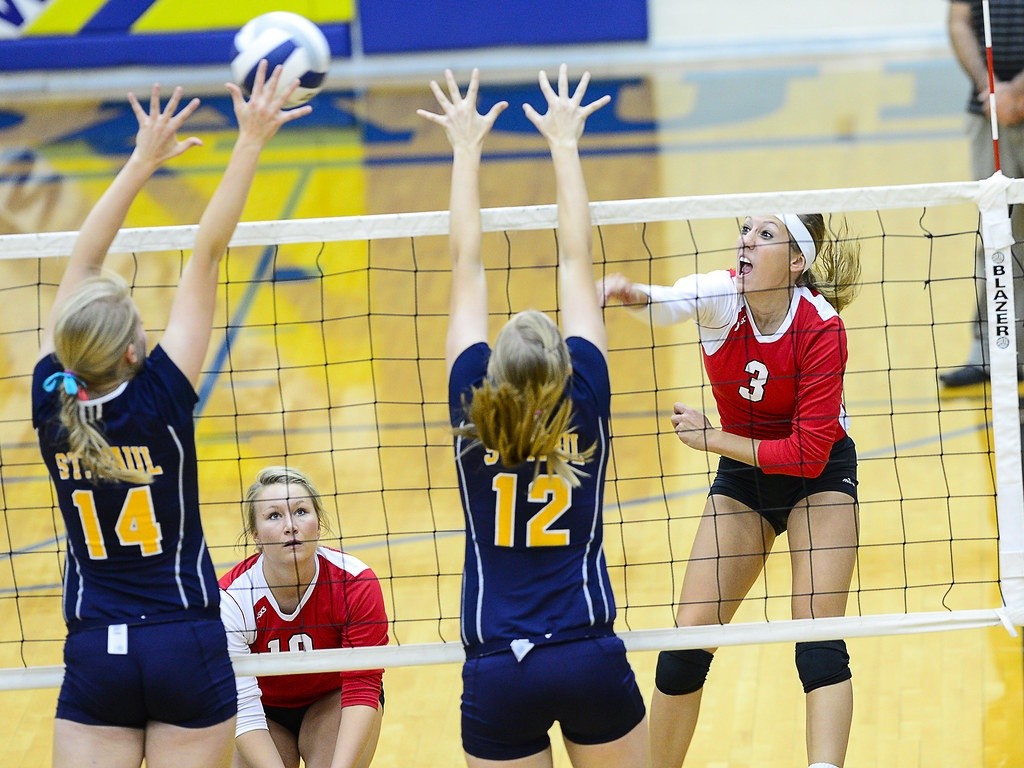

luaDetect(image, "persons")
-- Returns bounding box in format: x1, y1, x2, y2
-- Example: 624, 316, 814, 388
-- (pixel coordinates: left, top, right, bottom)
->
32, 60, 311, 768
416, 64, 648, 768
596, 212, 860, 767
940, 0, 1024, 387
218, 467, 389, 768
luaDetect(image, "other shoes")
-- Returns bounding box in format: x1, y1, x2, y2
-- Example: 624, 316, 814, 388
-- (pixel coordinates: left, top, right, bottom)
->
940, 365, 987, 386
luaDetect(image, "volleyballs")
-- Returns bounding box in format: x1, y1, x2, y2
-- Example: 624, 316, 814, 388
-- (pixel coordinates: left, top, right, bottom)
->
229, 11, 333, 109
